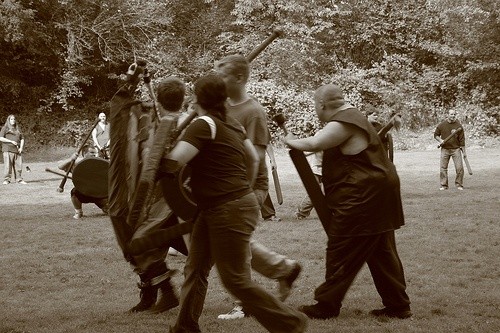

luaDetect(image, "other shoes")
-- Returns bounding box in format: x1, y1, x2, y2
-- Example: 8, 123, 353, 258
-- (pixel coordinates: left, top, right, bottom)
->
297, 303, 342, 320
369, 304, 414, 320
295, 212, 306, 221
455, 185, 463, 191
267, 259, 302, 304
18, 179, 27, 184
2, 178, 10, 185
215, 304, 250, 320
439, 186, 448, 191
263, 214, 281, 223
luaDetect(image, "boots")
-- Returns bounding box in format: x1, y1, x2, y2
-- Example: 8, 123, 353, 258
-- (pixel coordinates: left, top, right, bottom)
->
121, 285, 157, 314
144, 284, 181, 315
72, 208, 83, 220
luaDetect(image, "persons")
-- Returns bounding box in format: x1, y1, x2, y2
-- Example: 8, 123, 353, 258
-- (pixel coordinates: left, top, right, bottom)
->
59, 145, 108, 219
121, 55, 413, 333
434, 108, 466, 191
92, 112, 110, 159
0, 115, 27, 185
